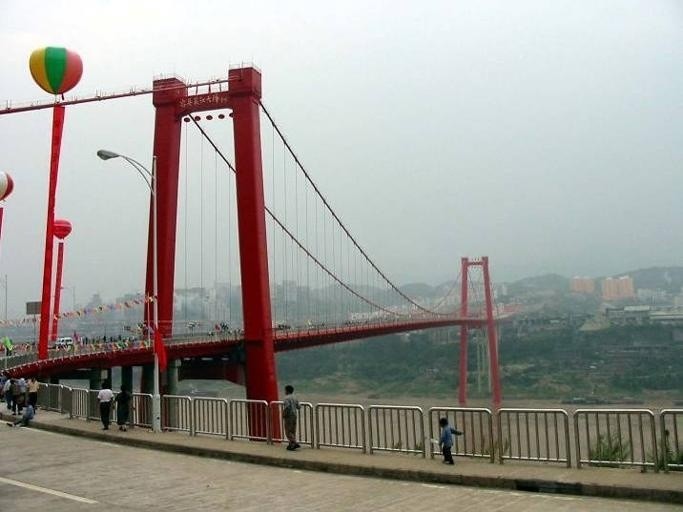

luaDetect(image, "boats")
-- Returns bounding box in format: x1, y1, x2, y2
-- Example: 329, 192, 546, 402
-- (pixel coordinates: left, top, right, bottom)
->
556, 382, 645, 406
670, 396, 683, 405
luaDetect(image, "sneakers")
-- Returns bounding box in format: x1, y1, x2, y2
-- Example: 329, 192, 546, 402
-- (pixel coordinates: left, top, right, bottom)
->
287, 444, 300, 450
7, 423, 12, 427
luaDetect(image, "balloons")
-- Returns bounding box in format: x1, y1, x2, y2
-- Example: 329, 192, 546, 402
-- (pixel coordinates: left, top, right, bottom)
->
54, 220, 72, 240
0, 172, 14, 203
30, 46, 83, 96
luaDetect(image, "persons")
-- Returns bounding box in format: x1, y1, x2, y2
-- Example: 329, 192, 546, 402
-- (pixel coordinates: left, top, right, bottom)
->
0, 370, 39, 427
439, 417, 464, 465
282, 385, 302, 450
80, 334, 125, 346
0, 333, 39, 356
113, 385, 131, 431
97, 382, 114, 429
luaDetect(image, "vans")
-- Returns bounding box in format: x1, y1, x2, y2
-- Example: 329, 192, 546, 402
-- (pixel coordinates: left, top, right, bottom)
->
55, 336, 73, 345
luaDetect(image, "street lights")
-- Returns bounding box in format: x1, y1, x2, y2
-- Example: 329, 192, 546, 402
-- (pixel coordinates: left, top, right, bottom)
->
98, 146, 163, 432
